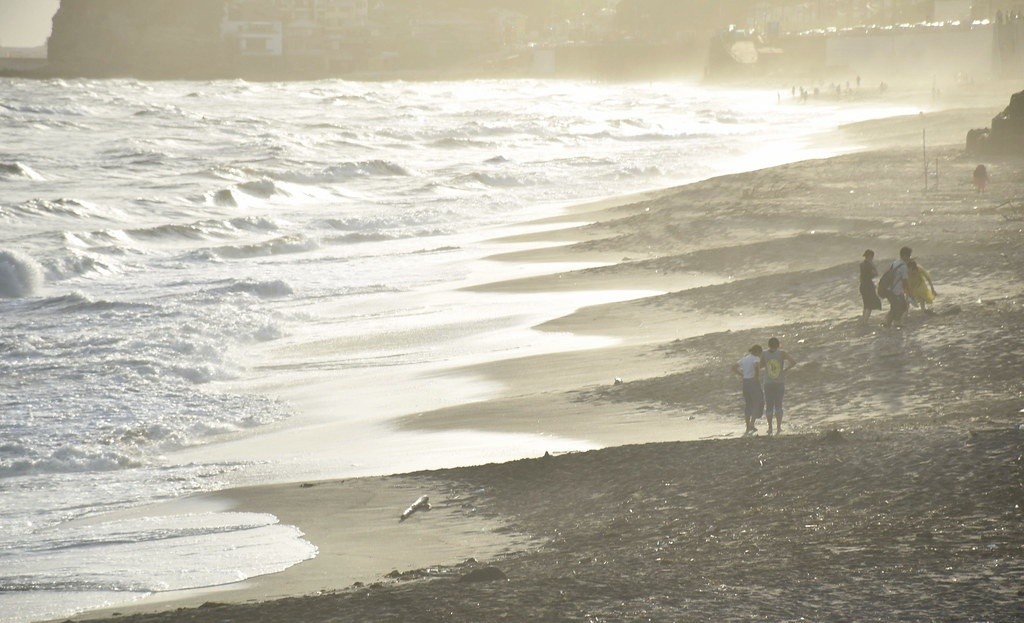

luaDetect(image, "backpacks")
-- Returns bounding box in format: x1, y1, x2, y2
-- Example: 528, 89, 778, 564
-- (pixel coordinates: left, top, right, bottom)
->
878, 263, 903, 298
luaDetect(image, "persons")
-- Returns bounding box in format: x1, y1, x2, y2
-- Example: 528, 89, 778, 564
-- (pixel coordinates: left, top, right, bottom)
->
973, 164, 987, 193
859, 250, 882, 323
883, 246, 913, 330
759, 337, 797, 433
903, 259, 937, 310
732, 344, 765, 433
